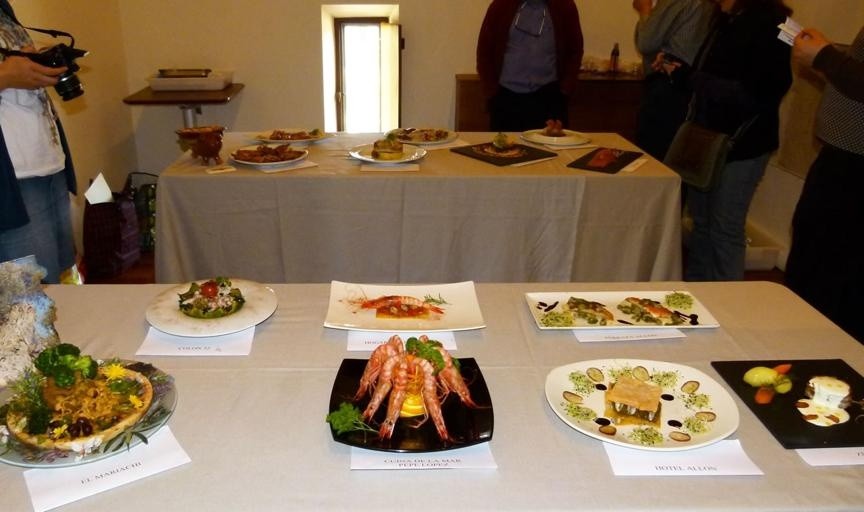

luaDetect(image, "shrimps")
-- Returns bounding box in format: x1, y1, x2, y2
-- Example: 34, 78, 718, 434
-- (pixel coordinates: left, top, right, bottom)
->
352, 334, 490, 443
346, 288, 446, 314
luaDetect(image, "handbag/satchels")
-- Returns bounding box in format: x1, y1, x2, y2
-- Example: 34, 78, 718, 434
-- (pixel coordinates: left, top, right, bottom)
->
82, 182, 140, 276
665, 117, 736, 192
137, 183, 157, 248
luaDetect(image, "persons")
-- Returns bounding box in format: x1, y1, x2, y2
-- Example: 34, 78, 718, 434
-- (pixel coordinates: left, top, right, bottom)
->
474, 1, 584, 132
787, 16, 864, 344
631, 0, 716, 219
650, 1, 793, 282
0, 0, 78, 284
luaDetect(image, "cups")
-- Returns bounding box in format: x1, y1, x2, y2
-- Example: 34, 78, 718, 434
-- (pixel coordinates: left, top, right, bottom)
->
174, 124, 225, 167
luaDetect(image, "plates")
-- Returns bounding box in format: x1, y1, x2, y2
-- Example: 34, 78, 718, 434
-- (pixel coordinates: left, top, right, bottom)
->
229, 143, 309, 168
1, 359, 178, 468
450, 141, 559, 167
323, 279, 488, 333
524, 288, 720, 332
384, 129, 458, 145
349, 143, 428, 163
543, 359, 741, 453
520, 129, 592, 146
253, 130, 341, 148
146, 278, 277, 338
329, 357, 494, 452
567, 146, 644, 174
711, 358, 864, 450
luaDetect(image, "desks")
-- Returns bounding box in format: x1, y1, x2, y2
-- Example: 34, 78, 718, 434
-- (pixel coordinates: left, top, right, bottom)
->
121, 82, 245, 130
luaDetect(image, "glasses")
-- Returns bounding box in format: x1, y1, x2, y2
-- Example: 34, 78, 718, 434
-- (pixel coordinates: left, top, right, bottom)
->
513, 1, 547, 38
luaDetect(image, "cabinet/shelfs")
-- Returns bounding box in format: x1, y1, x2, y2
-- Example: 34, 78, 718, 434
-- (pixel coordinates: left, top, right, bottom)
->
454, 71, 668, 143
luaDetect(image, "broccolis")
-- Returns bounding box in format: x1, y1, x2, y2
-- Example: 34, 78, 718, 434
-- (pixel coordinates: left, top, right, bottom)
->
27, 343, 98, 434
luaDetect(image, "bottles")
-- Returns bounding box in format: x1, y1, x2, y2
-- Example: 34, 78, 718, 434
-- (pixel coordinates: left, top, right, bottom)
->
609, 42, 620, 74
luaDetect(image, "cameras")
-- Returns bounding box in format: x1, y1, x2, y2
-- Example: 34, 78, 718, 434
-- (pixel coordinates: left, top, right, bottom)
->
41, 43, 84, 101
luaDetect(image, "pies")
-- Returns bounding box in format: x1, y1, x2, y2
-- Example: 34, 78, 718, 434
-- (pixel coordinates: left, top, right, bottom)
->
6, 365, 153, 451
